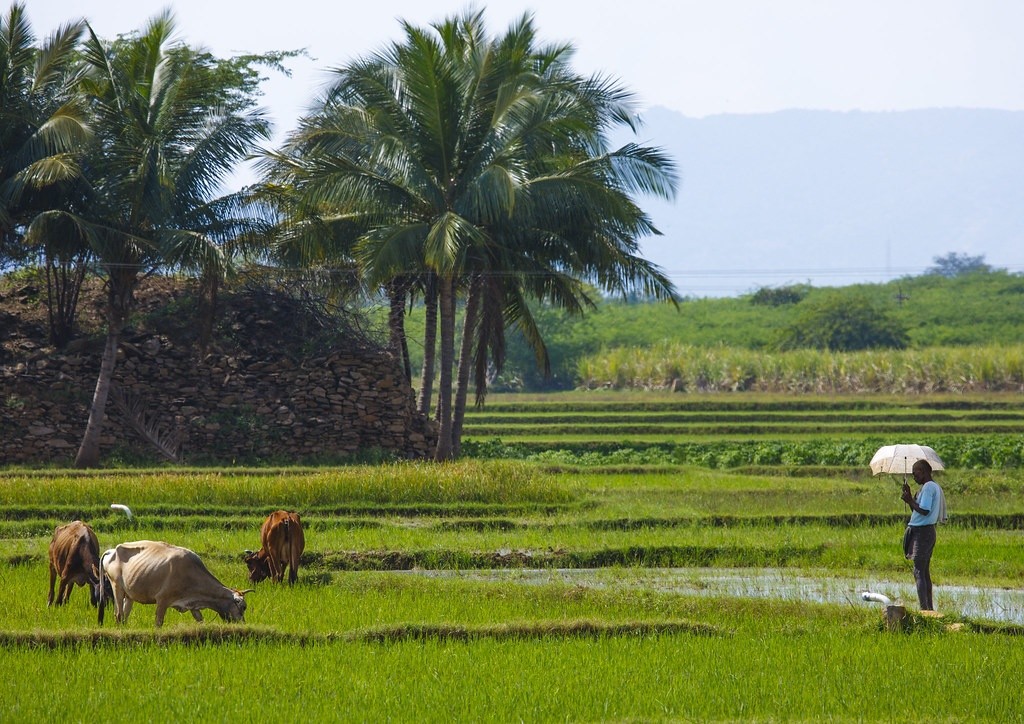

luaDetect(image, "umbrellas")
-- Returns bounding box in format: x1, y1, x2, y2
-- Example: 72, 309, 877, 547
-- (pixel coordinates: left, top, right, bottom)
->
868, 444, 947, 513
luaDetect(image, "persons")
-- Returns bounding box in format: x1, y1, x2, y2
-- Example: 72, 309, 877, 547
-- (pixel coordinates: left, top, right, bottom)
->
900, 459, 948, 611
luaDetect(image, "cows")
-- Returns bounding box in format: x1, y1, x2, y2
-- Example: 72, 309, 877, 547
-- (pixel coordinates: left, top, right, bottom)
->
94, 539, 256, 628
45, 518, 111, 611
239, 507, 307, 593
